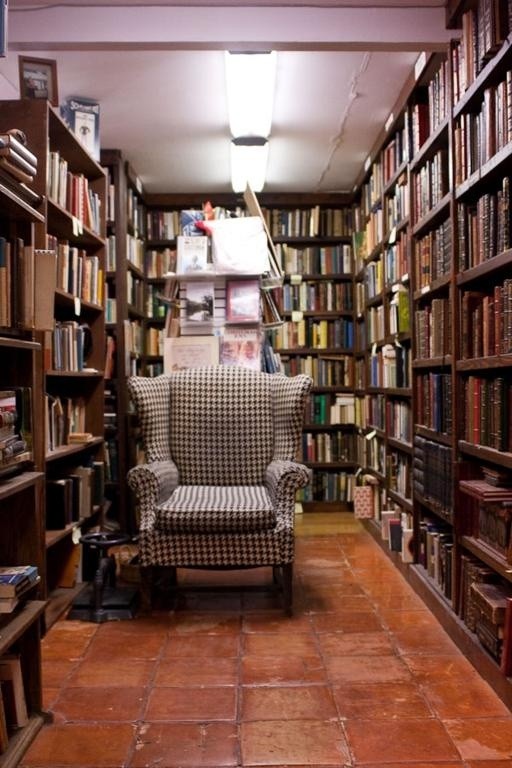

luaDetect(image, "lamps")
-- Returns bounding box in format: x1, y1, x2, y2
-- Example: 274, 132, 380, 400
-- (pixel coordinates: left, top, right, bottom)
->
227, 51, 275, 194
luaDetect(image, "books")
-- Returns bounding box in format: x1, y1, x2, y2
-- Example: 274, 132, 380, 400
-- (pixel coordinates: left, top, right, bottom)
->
0, 110, 148, 629
356, 0, 512, 668
148, 199, 354, 508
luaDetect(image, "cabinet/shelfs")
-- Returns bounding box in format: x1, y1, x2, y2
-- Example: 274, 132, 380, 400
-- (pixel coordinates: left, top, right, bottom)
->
145, 191, 361, 514
159, 270, 285, 373
353, 0, 512, 714
0, 100, 145, 640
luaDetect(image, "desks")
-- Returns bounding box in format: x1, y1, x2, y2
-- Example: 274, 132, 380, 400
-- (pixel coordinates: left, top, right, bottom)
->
0, 599, 50, 768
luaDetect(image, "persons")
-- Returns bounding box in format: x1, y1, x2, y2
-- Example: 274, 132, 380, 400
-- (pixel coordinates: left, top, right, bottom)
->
26, 77, 36, 99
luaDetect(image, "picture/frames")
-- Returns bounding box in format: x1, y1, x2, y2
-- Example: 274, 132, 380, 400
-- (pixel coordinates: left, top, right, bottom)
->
19, 54, 59, 110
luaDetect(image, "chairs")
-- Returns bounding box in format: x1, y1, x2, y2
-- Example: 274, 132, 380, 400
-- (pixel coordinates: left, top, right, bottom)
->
127, 365, 315, 620
